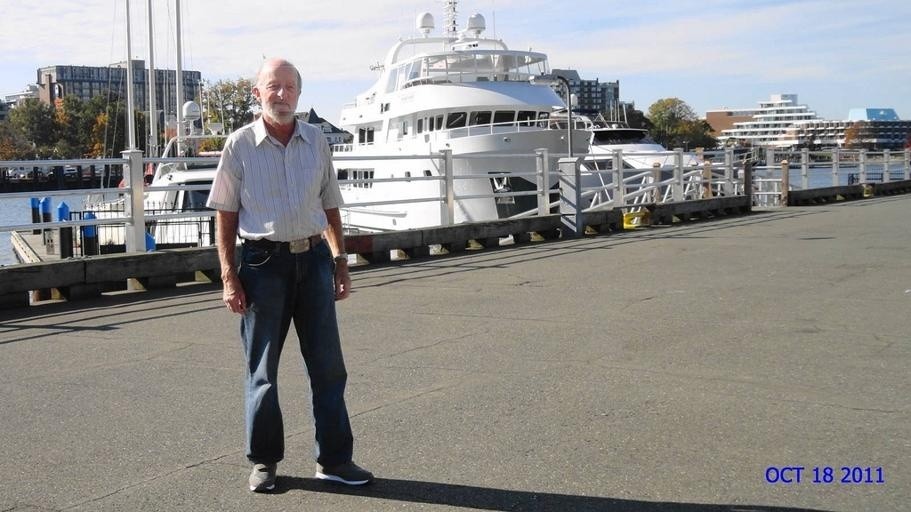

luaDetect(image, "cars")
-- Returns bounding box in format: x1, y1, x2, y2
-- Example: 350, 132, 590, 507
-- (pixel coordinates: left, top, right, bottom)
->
6, 167, 17, 178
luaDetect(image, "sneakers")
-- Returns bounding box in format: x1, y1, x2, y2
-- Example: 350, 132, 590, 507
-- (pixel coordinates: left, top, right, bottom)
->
315, 458, 374, 485
248, 459, 277, 492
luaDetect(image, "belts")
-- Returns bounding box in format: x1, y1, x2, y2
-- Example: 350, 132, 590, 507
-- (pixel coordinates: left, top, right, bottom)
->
245, 233, 326, 254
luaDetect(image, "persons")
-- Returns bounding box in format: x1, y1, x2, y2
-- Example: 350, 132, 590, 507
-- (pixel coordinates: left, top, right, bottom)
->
203, 57, 374, 494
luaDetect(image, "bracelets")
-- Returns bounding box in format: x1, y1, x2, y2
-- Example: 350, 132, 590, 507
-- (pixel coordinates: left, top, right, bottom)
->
331, 253, 348, 262
